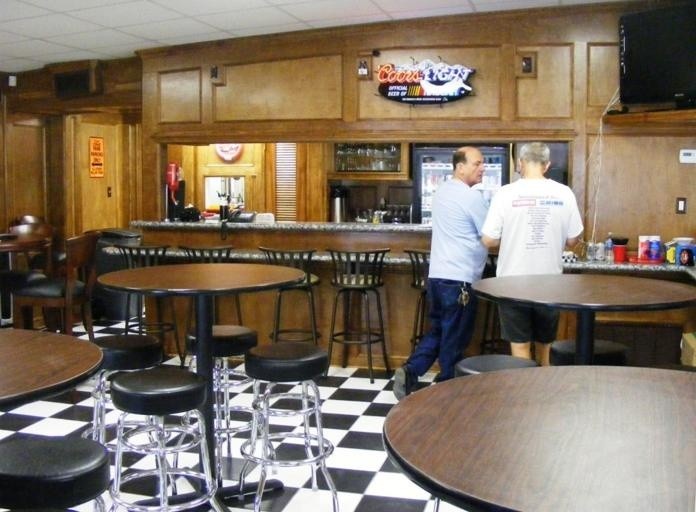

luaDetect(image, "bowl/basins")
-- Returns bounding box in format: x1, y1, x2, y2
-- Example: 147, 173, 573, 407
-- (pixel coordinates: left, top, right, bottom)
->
672, 237, 694, 244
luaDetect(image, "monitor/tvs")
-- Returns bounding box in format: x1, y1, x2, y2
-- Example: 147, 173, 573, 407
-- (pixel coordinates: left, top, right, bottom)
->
619, 0, 696, 111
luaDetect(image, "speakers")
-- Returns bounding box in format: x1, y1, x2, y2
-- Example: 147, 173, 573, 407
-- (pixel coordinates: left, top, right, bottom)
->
55, 70, 104, 99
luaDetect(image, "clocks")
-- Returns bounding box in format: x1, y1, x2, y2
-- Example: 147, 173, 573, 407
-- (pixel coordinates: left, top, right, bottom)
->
214, 143, 244, 164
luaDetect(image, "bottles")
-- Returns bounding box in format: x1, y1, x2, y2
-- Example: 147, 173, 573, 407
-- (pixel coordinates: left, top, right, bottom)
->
379, 197, 385, 209
364, 209, 384, 223
585, 232, 613, 264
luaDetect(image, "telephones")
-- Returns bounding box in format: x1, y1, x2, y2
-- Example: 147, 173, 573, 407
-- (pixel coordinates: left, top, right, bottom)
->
229, 209, 255, 222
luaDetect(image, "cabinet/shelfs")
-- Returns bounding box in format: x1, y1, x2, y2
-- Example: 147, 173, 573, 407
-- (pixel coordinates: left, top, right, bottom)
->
328, 142, 410, 182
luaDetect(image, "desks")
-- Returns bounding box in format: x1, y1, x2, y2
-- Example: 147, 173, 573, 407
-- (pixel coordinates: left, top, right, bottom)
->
96, 263, 306, 512
466, 273, 696, 365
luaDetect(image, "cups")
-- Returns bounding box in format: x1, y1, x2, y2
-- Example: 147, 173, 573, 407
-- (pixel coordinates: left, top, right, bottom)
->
638, 235, 649, 259
613, 245, 626, 263
649, 235, 660, 259
219, 194, 228, 222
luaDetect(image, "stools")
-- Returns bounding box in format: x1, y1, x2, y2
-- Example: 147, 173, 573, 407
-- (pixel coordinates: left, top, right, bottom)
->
0, 433, 109, 512
239, 343, 337, 511
81, 336, 176, 512
173, 324, 277, 470
109, 370, 223, 511
430, 354, 539, 510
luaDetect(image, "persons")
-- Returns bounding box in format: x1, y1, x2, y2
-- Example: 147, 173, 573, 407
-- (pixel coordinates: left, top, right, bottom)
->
481, 141, 584, 368
679, 249, 694, 266
392, 146, 489, 404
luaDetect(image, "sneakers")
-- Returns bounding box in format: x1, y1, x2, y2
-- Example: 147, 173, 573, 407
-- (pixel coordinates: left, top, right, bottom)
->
393, 365, 417, 401
